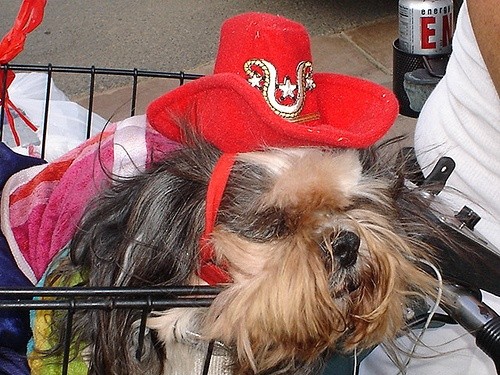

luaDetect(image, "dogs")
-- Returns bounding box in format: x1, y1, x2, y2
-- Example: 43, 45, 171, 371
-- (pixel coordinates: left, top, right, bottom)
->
20, 91, 500, 375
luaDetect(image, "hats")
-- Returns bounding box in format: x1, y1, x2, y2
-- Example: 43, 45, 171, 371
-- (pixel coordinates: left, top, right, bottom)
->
146, 12, 399, 154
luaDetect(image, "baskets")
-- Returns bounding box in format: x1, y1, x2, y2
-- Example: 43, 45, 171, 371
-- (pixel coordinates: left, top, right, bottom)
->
0, 63, 363, 374
393, 38, 424, 117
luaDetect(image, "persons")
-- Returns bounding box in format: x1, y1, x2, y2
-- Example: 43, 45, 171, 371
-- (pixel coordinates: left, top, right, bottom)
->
353, 0, 499, 375
